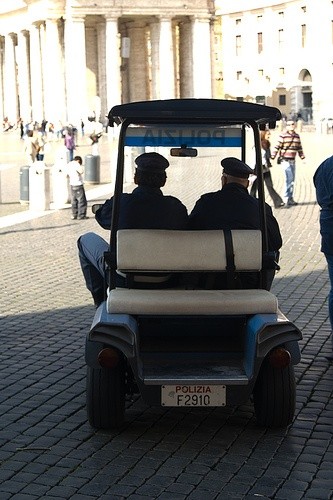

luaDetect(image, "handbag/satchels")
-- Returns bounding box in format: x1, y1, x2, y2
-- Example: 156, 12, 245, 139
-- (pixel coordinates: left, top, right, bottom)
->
277, 157, 281, 164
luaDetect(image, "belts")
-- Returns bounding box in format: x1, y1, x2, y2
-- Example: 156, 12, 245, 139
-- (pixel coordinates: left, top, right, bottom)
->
115, 269, 173, 283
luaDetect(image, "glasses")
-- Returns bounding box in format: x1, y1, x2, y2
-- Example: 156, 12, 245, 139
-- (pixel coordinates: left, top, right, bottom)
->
287, 123, 295, 126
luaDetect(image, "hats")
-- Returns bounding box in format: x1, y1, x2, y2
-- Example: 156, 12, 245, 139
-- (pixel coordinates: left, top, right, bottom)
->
135, 152, 169, 177
221, 157, 254, 178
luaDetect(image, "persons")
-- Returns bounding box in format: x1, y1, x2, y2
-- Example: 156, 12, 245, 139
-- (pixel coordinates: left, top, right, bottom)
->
62, 128, 75, 161
249, 129, 285, 208
80, 111, 110, 136
271, 119, 305, 205
64, 156, 89, 220
77, 152, 189, 307
190, 157, 282, 289
279, 112, 304, 132
23, 129, 45, 163
3, 116, 55, 139
314, 155, 333, 328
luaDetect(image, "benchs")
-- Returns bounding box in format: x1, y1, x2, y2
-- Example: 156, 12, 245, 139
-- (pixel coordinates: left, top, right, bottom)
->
105, 228, 279, 314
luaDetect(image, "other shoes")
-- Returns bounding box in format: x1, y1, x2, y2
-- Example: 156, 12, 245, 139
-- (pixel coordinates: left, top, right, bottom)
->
72, 216, 77, 220
275, 203, 285, 207
287, 201, 298, 205
78, 216, 89, 220
